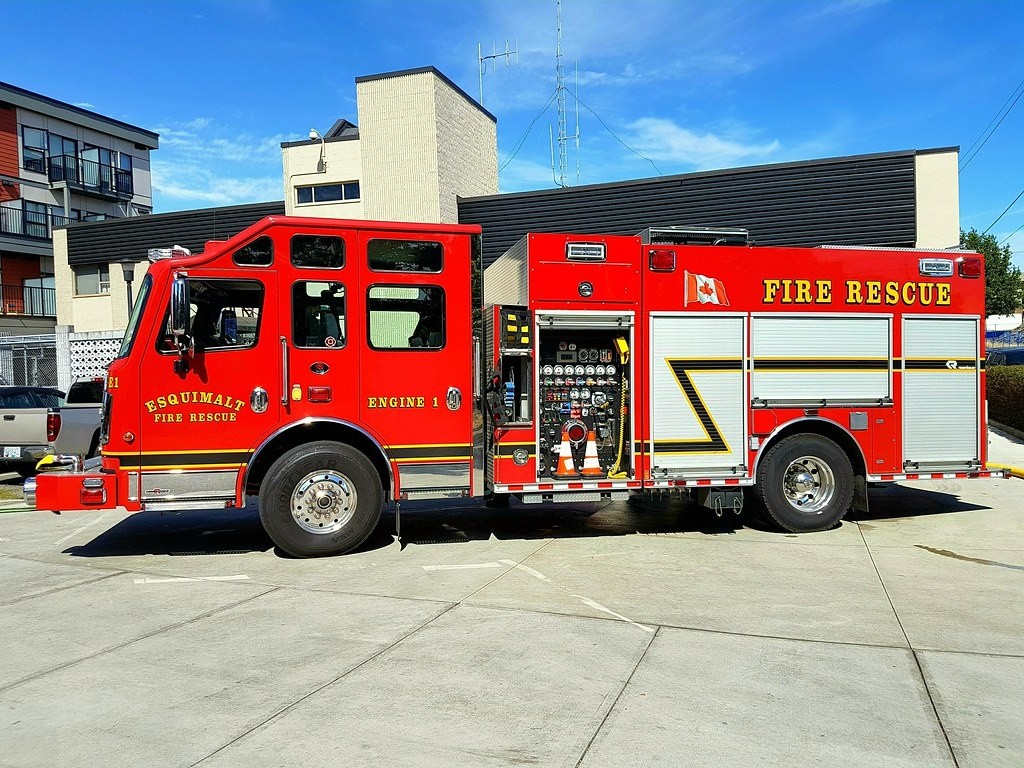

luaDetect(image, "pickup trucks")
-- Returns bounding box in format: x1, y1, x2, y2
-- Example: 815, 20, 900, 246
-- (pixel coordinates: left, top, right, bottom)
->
0, 377, 109, 478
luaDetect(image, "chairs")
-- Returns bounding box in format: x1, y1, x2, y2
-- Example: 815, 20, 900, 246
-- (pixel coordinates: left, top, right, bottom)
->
7, 303, 25, 315
306, 291, 345, 346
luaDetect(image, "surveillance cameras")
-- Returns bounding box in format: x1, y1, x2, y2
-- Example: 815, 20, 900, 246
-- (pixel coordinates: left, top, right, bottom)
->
308, 132, 317, 141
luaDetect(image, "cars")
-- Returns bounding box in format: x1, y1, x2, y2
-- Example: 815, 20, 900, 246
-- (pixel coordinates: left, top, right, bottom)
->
985, 348, 1024, 366
0, 385, 67, 411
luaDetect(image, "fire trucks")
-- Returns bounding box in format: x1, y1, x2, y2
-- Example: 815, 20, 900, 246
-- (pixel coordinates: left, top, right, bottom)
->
23, 214, 1012, 559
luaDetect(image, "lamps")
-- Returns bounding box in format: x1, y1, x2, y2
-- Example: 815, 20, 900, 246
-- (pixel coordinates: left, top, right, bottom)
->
2, 180, 15, 187
138, 208, 150, 214
